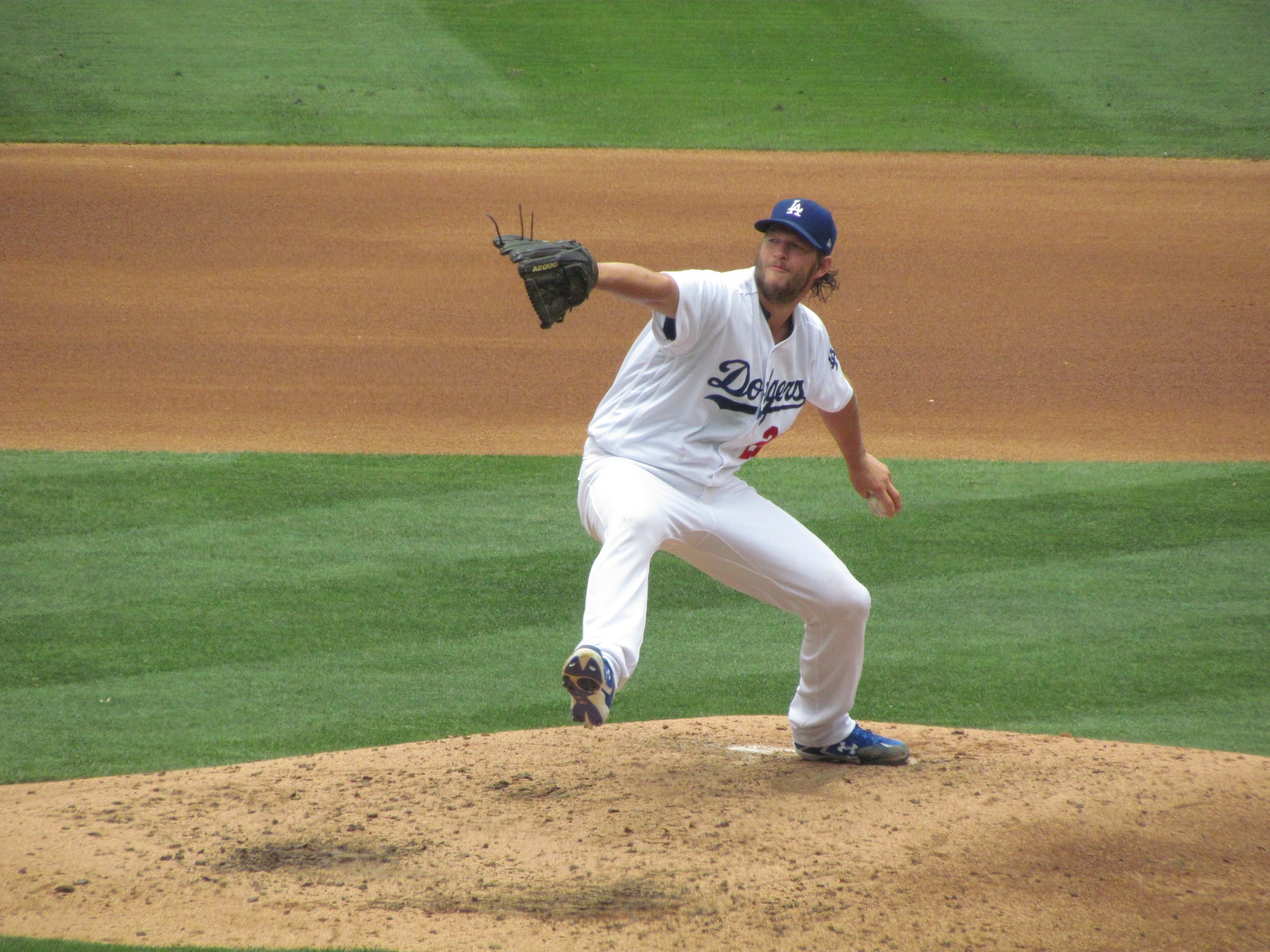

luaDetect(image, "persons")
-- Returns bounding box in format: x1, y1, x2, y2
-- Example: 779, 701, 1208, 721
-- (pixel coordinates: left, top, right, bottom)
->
482, 196, 904, 766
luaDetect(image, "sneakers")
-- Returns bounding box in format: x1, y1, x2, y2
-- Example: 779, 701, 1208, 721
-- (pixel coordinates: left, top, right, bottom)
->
794, 724, 909, 765
563, 645, 614, 729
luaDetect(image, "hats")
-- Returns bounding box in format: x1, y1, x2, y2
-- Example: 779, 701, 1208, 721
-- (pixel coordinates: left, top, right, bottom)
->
755, 198, 837, 254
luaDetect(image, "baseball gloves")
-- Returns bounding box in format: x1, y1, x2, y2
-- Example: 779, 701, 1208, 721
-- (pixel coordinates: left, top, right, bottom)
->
485, 203, 600, 331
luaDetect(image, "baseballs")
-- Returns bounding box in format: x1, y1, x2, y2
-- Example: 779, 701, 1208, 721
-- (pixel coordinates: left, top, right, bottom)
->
868, 493, 896, 518
